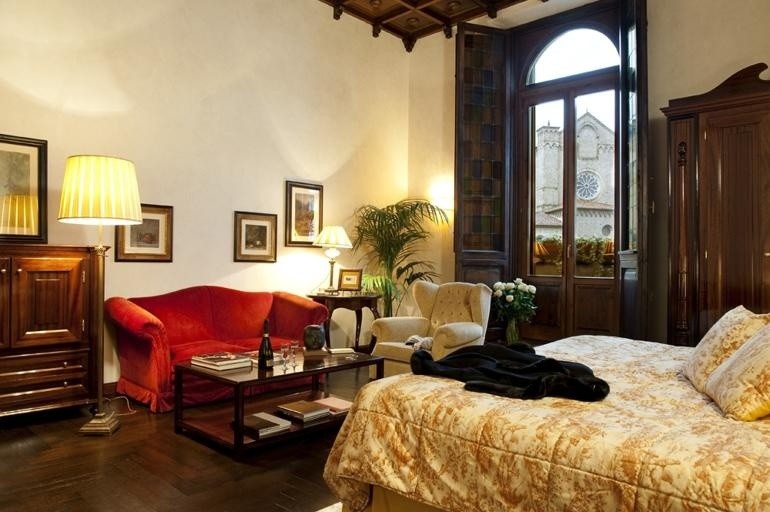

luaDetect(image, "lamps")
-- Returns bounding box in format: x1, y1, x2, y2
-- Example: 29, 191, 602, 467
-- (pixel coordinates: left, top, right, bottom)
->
312, 225, 353, 295
56, 155, 144, 437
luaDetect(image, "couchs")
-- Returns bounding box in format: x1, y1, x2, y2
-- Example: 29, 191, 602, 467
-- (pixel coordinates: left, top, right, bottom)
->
103, 285, 329, 414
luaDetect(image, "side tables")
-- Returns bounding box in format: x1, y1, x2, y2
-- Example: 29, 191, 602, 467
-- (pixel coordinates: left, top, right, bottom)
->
308, 293, 381, 356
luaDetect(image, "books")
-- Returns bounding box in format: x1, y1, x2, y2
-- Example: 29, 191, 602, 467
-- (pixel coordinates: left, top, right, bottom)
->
276, 399, 330, 418
312, 396, 353, 414
192, 351, 250, 365
232, 410, 292, 432
230, 425, 290, 438
190, 360, 251, 371
274, 412, 330, 423
328, 347, 355, 353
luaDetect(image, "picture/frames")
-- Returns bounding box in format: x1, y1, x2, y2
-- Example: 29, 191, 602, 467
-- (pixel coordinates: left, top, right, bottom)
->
115, 203, 173, 263
0, 134, 48, 245
232, 179, 325, 264
338, 268, 362, 291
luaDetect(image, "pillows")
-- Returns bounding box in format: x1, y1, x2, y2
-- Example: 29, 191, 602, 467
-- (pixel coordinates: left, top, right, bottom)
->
682, 303, 770, 420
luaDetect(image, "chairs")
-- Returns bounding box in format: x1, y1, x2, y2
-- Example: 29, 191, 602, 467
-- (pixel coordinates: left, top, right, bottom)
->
368, 279, 493, 379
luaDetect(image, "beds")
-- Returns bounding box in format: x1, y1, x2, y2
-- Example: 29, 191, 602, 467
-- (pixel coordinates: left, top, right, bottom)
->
352, 332, 770, 512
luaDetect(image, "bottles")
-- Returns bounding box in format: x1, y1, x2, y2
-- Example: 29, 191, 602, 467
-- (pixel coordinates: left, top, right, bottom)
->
258, 369, 273, 379
259, 320, 274, 369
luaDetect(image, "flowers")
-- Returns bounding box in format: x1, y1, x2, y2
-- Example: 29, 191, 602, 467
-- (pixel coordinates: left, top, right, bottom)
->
493, 277, 539, 345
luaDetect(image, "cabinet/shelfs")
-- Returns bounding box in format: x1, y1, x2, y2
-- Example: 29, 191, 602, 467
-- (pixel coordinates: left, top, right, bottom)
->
661, 89, 769, 347
0, 247, 97, 418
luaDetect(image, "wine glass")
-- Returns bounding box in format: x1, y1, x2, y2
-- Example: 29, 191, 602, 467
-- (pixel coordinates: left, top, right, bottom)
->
289, 339, 298, 367
280, 343, 290, 370
